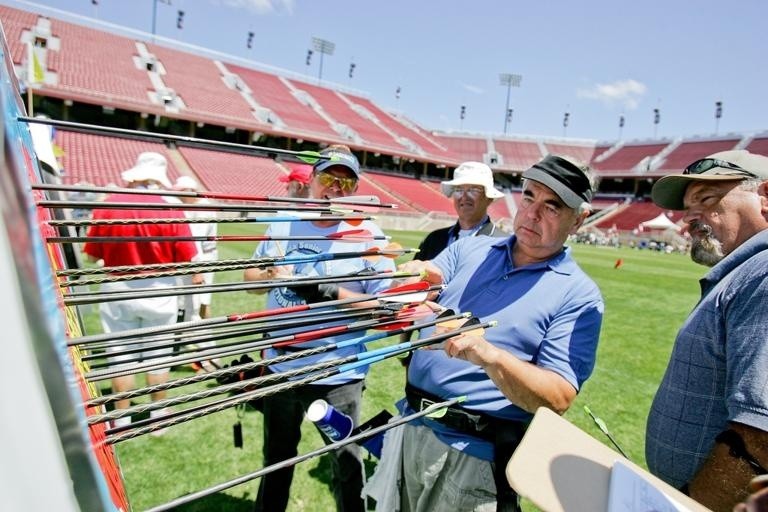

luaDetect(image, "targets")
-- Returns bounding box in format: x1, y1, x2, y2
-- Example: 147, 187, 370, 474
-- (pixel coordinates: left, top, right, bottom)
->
0, 20, 132, 512
30, 112, 83, 280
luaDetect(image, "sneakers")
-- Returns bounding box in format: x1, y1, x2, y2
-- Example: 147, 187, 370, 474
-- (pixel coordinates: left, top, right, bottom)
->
110, 406, 173, 435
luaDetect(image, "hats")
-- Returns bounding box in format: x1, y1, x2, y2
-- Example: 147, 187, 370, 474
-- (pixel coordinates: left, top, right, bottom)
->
174, 176, 198, 192
121, 151, 172, 189
441, 161, 505, 201
279, 165, 313, 184
521, 153, 593, 210
27, 116, 59, 176
313, 146, 360, 178
652, 150, 768, 210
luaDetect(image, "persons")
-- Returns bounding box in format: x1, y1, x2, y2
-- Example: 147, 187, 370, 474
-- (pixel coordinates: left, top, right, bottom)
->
244, 142, 398, 512
643, 149, 767, 512
400, 161, 512, 368
171, 176, 217, 371
570, 223, 688, 256
731, 472, 768, 512
81, 152, 197, 440
277, 164, 322, 209
359, 153, 604, 511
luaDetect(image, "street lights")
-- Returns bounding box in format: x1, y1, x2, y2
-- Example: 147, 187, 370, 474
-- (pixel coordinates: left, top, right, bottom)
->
653, 108, 660, 139
564, 111, 570, 139
713, 100, 724, 137
507, 108, 514, 132
617, 115, 625, 140
458, 104, 466, 130
499, 72, 523, 134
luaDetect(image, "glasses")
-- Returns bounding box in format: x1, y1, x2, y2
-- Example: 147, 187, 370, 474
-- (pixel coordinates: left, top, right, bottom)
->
682, 158, 757, 178
452, 186, 483, 198
317, 171, 358, 194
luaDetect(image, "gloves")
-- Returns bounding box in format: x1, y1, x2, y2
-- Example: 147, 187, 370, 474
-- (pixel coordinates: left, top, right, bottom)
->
287, 266, 340, 302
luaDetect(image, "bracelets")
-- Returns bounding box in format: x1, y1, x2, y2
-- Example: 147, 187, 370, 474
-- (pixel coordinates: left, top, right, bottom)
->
678, 482, 691, 499
265, 266, 272, 280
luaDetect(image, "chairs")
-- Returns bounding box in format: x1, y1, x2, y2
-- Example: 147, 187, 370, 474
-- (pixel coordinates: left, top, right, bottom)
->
0, 2, 764, 233
